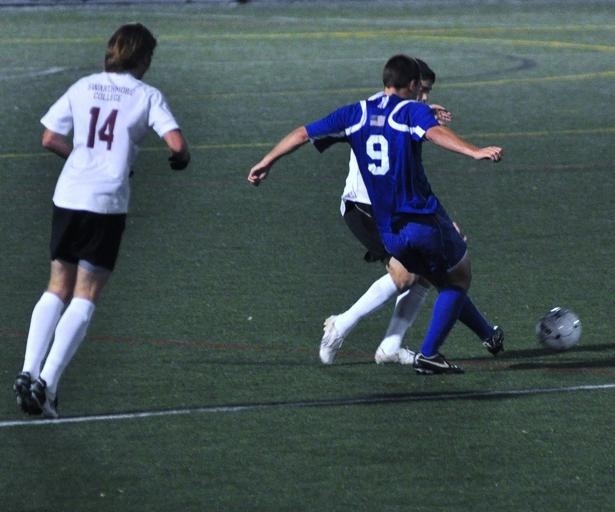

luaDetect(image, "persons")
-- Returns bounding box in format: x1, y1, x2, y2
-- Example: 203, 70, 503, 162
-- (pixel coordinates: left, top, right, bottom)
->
248, 55, 504, 374
319, 62, 454, 367
13, 23, 191, 418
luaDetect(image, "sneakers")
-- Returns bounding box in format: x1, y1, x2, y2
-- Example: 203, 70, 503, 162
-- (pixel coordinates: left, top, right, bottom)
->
375, 347, 464, 374
13, 372, 58, 418
319, 315, 344, 365
483, 326, 504, 355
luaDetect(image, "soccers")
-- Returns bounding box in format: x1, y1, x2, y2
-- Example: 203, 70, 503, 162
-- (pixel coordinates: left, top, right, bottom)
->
536, 307, 582, 351
